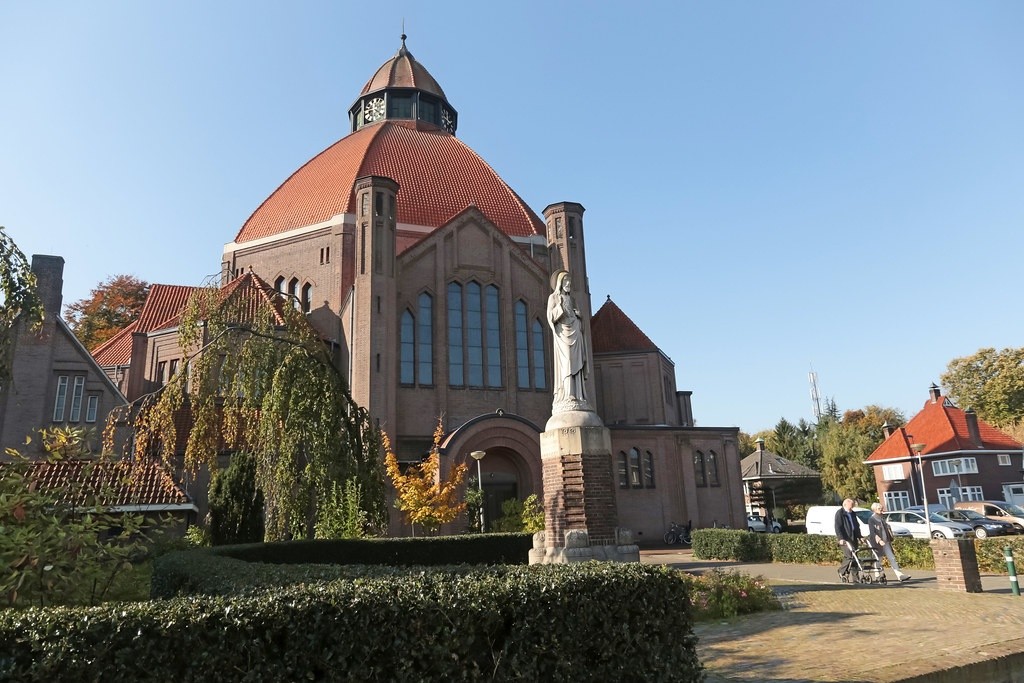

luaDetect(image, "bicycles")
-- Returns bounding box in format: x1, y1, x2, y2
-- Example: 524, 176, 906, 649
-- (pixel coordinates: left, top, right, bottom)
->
713, 520, 731, 529
664, 522, 694, 544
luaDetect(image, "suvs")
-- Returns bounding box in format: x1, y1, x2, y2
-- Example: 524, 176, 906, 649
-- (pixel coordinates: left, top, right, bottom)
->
881, 510, 975, 539
747, 515, 782, 534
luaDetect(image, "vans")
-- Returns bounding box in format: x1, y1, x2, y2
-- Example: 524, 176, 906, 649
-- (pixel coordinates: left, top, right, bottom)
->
954, 501, 1024, 529
904, 503, 946, 520
805, 506, 874, 537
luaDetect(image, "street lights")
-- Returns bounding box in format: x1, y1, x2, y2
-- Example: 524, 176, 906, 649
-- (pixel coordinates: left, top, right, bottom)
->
471, 451, 486, 532
950, 462, 963, 501
911, 444, 932, 539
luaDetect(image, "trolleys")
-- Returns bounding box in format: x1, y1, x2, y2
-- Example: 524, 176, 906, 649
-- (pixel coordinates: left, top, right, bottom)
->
839, 539, 886, 585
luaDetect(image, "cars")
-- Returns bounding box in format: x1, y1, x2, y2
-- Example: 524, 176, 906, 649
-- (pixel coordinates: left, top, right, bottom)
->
937, 509, 1015, 540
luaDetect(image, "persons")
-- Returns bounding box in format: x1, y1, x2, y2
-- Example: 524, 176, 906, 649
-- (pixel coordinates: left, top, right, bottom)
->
835, 499, 867, 583
547, 269, 593, 412
868, 503, 911, 581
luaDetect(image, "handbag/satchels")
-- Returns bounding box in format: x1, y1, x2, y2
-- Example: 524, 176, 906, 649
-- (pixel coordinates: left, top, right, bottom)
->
883, 521, 893, 541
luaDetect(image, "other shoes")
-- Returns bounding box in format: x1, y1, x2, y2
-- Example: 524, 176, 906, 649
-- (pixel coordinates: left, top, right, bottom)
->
900, 575, 911, 582
